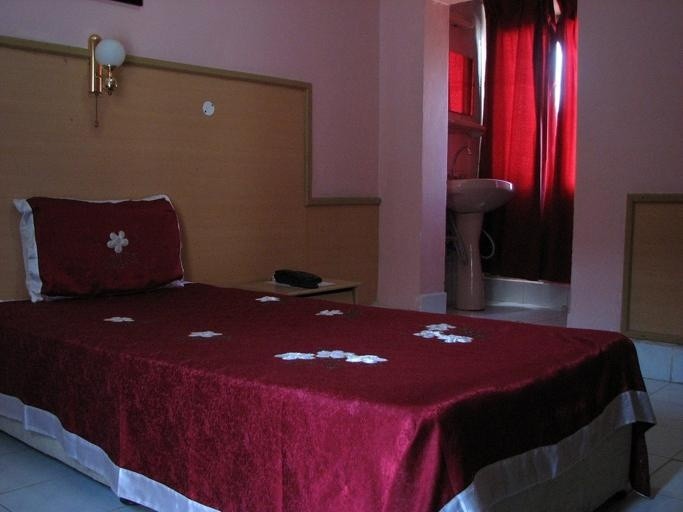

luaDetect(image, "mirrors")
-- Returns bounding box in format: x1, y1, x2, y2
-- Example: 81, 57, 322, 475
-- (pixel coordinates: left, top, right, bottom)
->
447, 42, 478, 115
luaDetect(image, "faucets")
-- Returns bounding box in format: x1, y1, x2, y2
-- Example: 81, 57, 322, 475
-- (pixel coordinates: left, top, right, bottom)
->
451, 146, 473, 177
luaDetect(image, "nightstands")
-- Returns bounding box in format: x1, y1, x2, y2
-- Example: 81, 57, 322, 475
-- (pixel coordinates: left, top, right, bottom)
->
234, 279, 361, 307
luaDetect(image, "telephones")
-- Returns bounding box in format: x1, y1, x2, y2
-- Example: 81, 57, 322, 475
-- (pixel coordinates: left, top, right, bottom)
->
273, 268, 324, 289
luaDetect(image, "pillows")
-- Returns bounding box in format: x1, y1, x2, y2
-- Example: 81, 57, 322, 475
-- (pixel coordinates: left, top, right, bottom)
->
11, 190, 186, 302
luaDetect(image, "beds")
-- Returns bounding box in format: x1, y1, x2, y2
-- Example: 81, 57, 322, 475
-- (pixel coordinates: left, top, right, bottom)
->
0, 285, 636, 511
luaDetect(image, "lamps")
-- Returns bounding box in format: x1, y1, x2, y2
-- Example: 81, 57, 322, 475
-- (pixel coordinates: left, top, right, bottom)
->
87, 32, 127, 95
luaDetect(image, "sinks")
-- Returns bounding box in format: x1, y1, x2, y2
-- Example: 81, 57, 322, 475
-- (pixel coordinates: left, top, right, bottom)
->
446, 178, 516, 212
